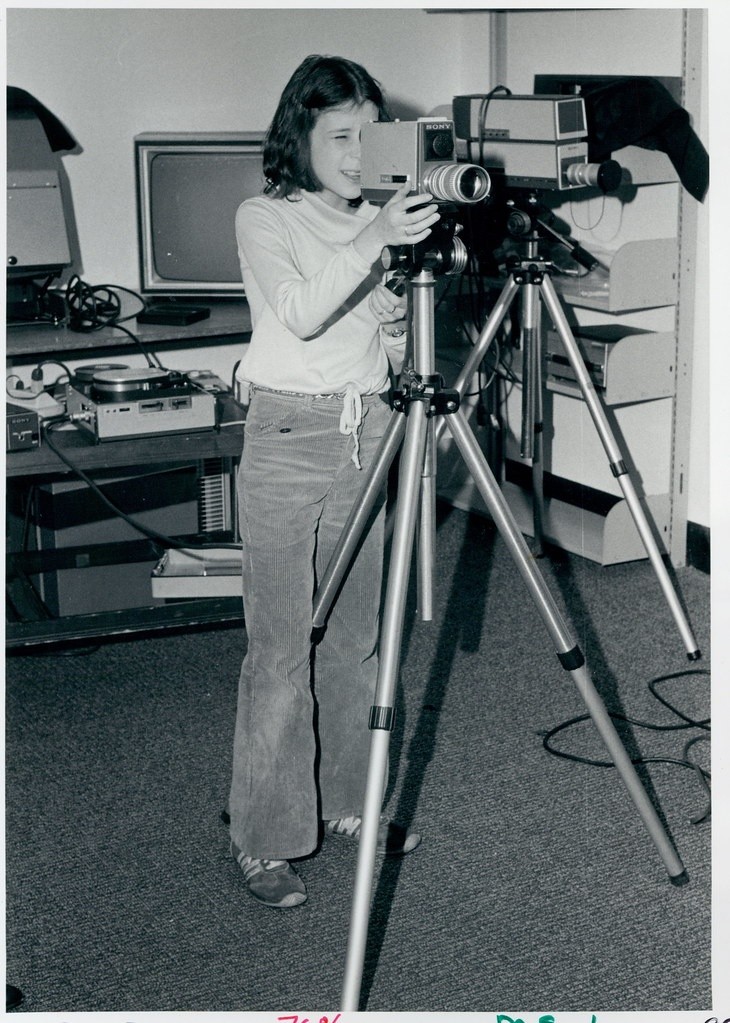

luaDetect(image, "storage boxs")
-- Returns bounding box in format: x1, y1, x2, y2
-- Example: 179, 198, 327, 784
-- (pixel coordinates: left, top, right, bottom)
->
7, 401, 39, 452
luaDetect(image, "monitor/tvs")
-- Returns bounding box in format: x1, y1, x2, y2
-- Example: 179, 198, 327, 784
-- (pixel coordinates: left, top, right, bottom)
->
135, 132, 275, 300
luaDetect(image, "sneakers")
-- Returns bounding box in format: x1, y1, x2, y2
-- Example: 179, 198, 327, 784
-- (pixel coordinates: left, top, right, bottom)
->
230, 838, 307, 907
319, 813, 421, 854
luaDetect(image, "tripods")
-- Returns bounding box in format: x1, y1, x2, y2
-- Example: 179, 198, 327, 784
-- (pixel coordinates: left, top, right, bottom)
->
220, 212, 693, 1012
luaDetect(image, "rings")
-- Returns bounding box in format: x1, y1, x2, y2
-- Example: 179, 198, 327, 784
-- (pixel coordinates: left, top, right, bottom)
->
405, 227, 409, 235
389, 307, 396, 314
379, 310, 385, 315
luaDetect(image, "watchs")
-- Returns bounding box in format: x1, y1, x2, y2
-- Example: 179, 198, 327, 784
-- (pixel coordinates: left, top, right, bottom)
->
382, 326, 407, 337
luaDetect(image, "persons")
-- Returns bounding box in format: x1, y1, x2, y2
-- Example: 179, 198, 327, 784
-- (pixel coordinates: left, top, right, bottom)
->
226, 55, 441, 907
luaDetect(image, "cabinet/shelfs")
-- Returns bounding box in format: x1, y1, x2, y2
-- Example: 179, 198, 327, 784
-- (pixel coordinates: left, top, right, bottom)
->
505, 144, 701, 571
6, 290, 254, 654
